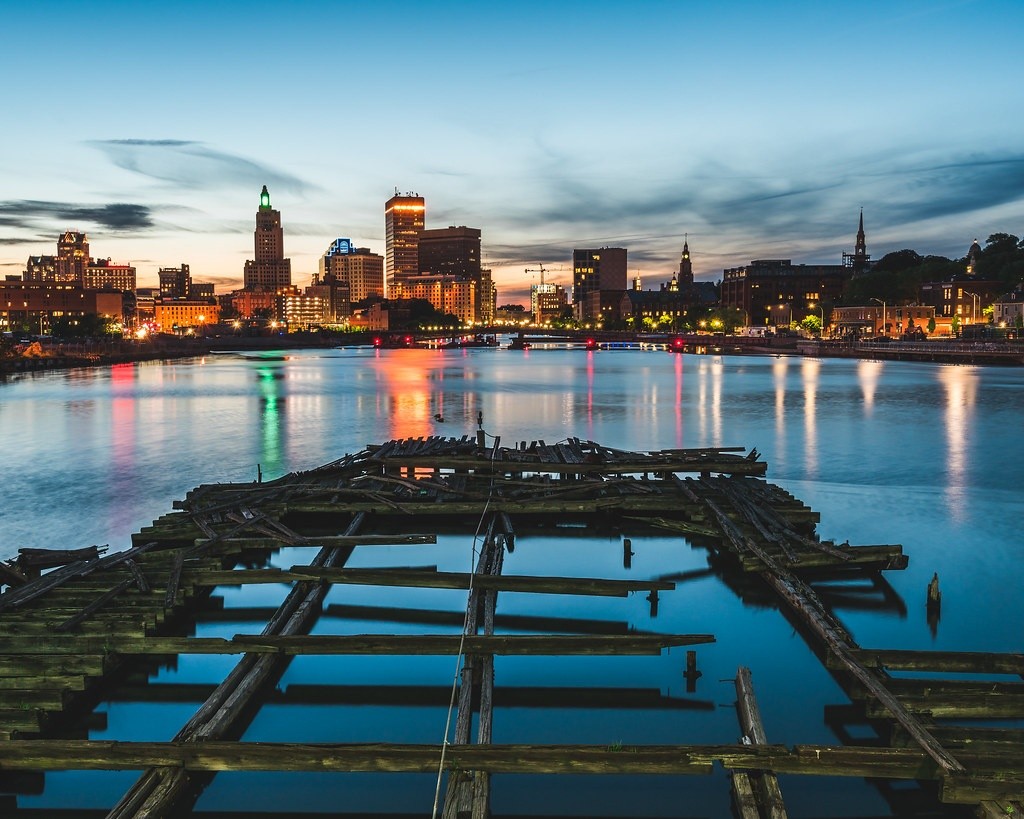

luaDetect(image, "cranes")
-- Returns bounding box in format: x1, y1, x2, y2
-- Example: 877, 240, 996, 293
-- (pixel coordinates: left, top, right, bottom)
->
524, 262, 574, 284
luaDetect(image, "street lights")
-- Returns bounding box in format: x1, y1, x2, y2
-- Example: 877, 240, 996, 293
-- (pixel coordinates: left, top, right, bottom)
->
780, 305, 792, 322
959, 290, 977, 325
736, 307, 748, 328
971, 291, 981, 322
809, 304, 824, 337
40, 314, 48, 336
105, 314, 109, 343
869, 297, 886, 337
